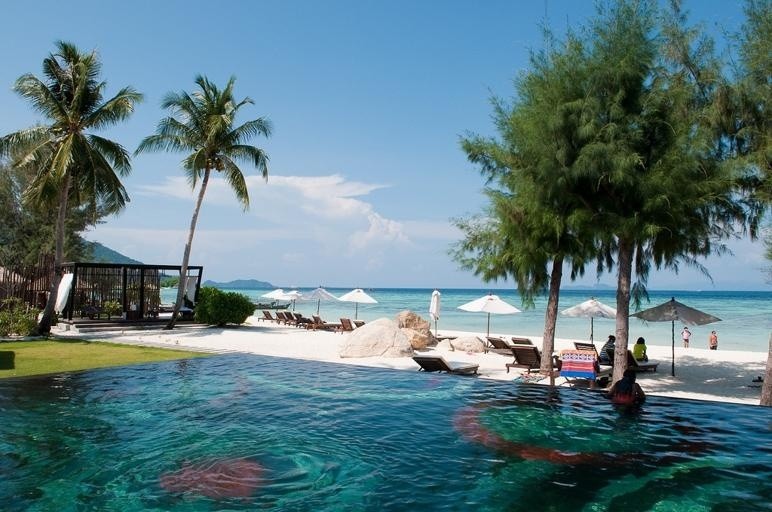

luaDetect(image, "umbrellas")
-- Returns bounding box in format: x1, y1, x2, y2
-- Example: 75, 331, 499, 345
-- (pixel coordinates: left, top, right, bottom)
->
456, 291, 522, 347
625, 294, 723, 378
301, 286, 339, 315
557, 296, 616, 345
263, 288, 289, 310
428, 287, 441, 338
281, 289, 310, 312
338, 288, 379, 320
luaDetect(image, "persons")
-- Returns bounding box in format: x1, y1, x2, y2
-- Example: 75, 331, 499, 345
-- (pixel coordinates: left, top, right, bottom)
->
605, 365, 649, 410
680, 326, 691, 348
632, 336, 648, 362
599, 334, 615, 361
709, 331, 718, 350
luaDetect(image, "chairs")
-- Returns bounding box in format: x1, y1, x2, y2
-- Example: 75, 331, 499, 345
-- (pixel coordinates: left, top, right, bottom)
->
410, 354, 483, 376
485, 334, 662, 389
257, 310, 367, 335
